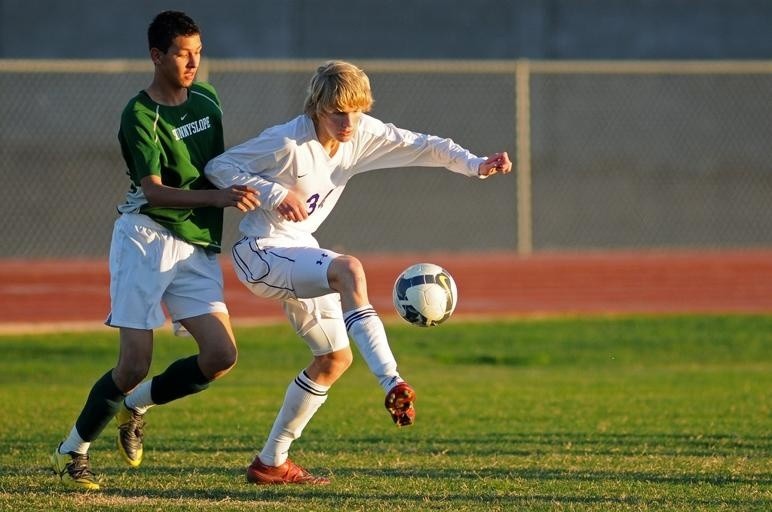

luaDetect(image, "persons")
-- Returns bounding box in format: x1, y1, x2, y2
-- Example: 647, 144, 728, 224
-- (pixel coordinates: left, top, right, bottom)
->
50, 11, 261, 492
203, 60, 512, 485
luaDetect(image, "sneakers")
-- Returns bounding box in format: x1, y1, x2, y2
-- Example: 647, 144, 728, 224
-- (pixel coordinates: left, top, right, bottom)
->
247, 455, 331, 484
51, 441, 100, 490
385, 382, 416, 427
115, 399, 146, 467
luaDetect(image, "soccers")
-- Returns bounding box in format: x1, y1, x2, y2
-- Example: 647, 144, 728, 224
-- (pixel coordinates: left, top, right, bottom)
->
392, 263, 457, 327
